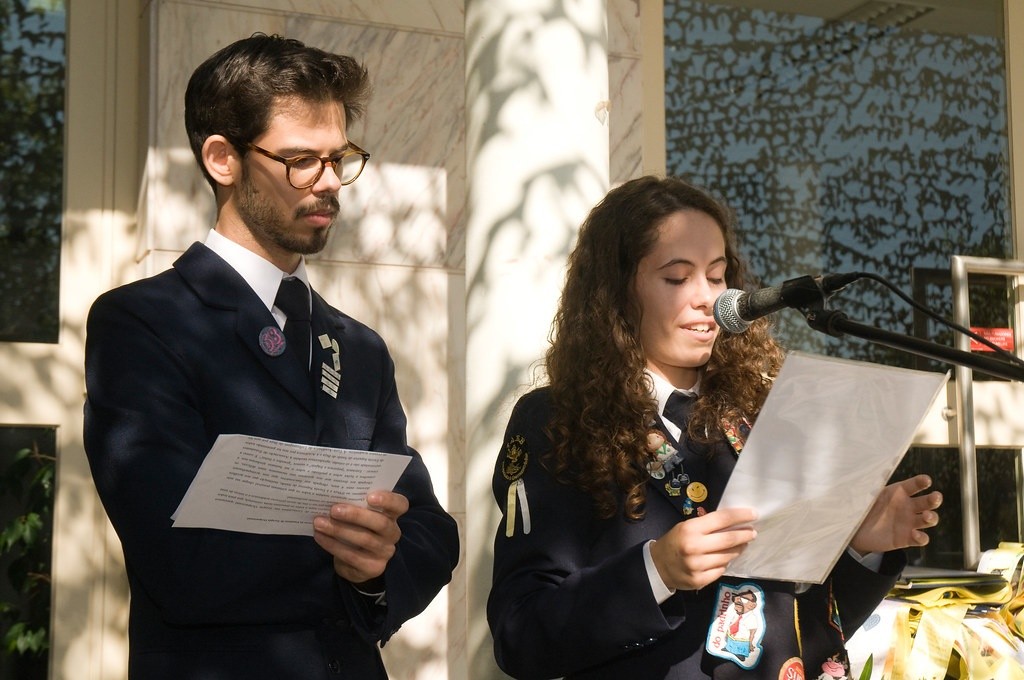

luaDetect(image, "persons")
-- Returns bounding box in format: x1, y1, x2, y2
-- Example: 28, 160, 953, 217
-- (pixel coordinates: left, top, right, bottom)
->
485, 175, 942, 680
81, 32, 459, 678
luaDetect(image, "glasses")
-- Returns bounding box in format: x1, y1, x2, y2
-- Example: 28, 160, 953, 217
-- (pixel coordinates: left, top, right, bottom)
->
222, 130, 371, 189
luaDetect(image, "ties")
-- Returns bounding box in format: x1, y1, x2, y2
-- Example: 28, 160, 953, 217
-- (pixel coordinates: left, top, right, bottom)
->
663, 389, 713, 480
274, 277, 311, 378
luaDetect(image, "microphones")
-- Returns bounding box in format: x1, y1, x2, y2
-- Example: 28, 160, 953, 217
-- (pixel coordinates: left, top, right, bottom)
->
715, 271, 859, 334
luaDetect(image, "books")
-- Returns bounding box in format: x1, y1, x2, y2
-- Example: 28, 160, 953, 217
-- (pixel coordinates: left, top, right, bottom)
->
894, 566, 1008, 590
